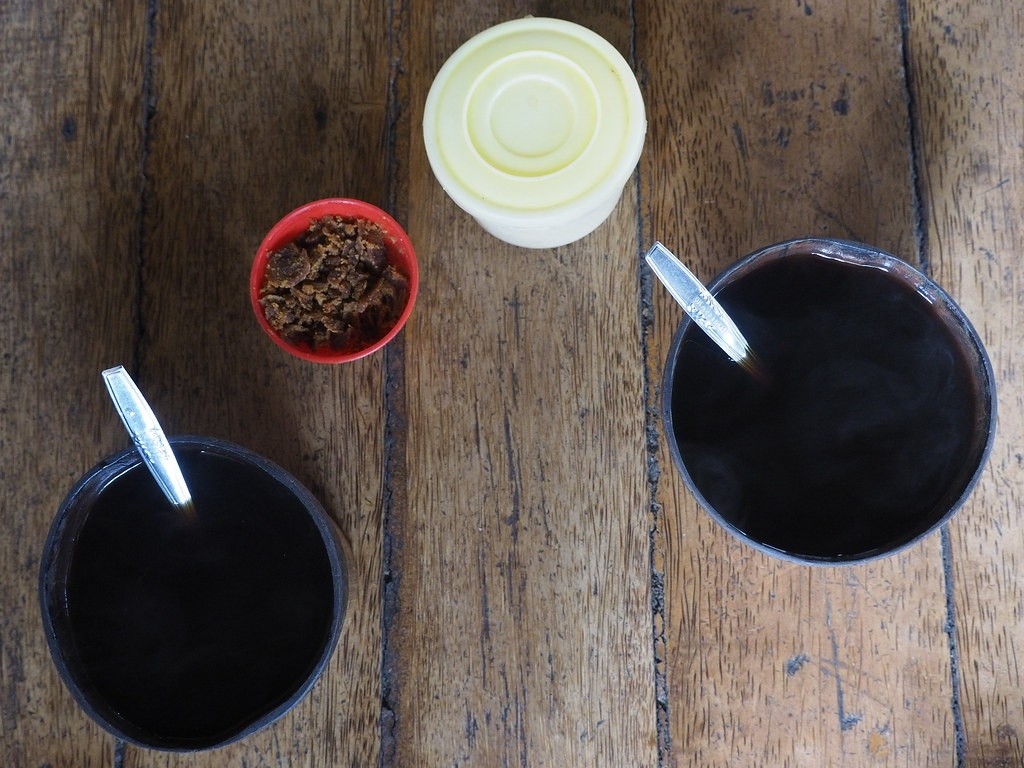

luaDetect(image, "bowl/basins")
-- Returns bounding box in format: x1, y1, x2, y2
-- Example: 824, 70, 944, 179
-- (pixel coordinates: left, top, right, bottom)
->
248, 197, 419, 365
35, 434, 350, 755
659, 235, 998, 569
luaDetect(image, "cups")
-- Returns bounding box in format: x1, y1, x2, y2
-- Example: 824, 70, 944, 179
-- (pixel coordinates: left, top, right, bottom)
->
422, 16, 648, 251
